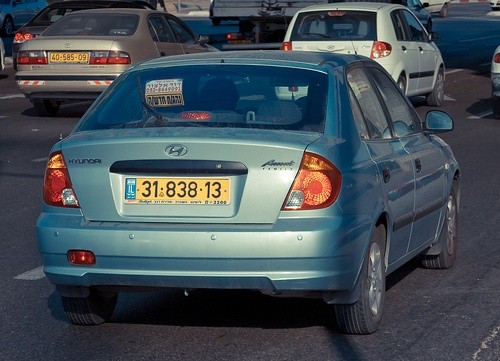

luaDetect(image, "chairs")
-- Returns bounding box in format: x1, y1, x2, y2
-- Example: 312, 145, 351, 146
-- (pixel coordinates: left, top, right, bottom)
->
257, 100, 302, 123
309, 21, 327, 36
109, 28, 130, 35
64, 29, 81, 35
198, 78, 242, 110
357, 21, 369, 35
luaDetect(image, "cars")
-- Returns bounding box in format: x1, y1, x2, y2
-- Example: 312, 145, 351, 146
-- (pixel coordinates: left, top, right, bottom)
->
0, 0, 49, 44
16, 8, 222, 113
389, 0, 434, 34
33, 50, 461, 335
280, 2, 446, 108
13, 0, 157, 69
419, 0, 451, 18
490, 45, 500, 117
162, 1, 330, 51
